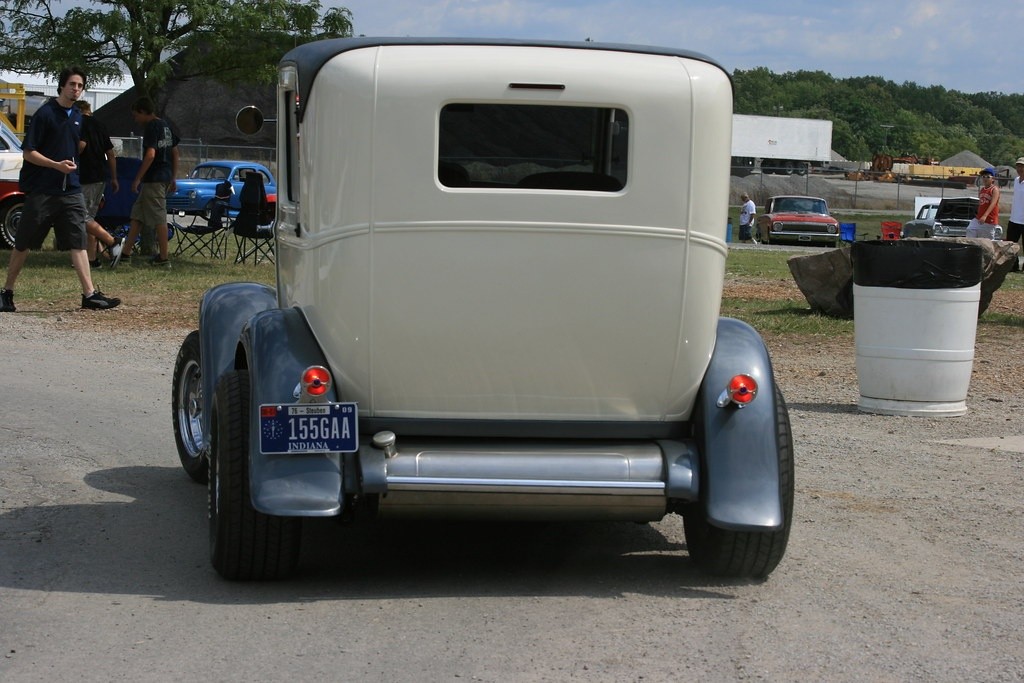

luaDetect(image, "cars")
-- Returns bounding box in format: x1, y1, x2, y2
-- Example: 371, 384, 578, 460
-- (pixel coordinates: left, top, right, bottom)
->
755, 196, 840, 248
171, 35, 795, 583
932, 197, 1002, 240
0, 121, 26, 250
166, 161, 277, 220
903, 203, 939, 238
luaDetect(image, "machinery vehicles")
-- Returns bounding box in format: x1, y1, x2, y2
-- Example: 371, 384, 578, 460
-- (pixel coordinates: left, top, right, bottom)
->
845, 153, 1015, 188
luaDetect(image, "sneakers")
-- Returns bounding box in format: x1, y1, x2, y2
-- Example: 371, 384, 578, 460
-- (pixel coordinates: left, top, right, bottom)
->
101, 236, 126, 268
116, 253, 130, 266
81, 284, 121, 309
0, 287, 17, 312
145, 254, 171, 269
71, 257, 103, 268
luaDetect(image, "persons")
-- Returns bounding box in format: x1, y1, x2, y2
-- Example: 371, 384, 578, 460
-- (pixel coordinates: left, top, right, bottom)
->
738, 192, 758, 244
110, 98, 180, 266
1006, 158, 1024, 273
71, 100, 125, 269
0, 67, 122, 311
966, 167, 1000, 240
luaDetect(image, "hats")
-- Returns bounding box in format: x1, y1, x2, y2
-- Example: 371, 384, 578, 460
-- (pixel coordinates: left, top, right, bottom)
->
980, 167, 995, 176
1015, 157, 1024, 166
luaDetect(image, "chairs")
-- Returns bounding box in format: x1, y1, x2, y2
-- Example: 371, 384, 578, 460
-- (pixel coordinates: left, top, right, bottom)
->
95, 157, 175, 256
228, 171, 276, 266
838, 222, 869, 248
171, 180, 234, 260
875, 221, 905, 240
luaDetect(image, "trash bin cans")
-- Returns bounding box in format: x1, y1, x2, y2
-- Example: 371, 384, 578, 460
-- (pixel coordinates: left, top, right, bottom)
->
726, 218, 733, 243
850, 238, 983, 416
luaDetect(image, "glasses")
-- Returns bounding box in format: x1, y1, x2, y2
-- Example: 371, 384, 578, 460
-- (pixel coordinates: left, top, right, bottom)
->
982, 175, 993, 178
